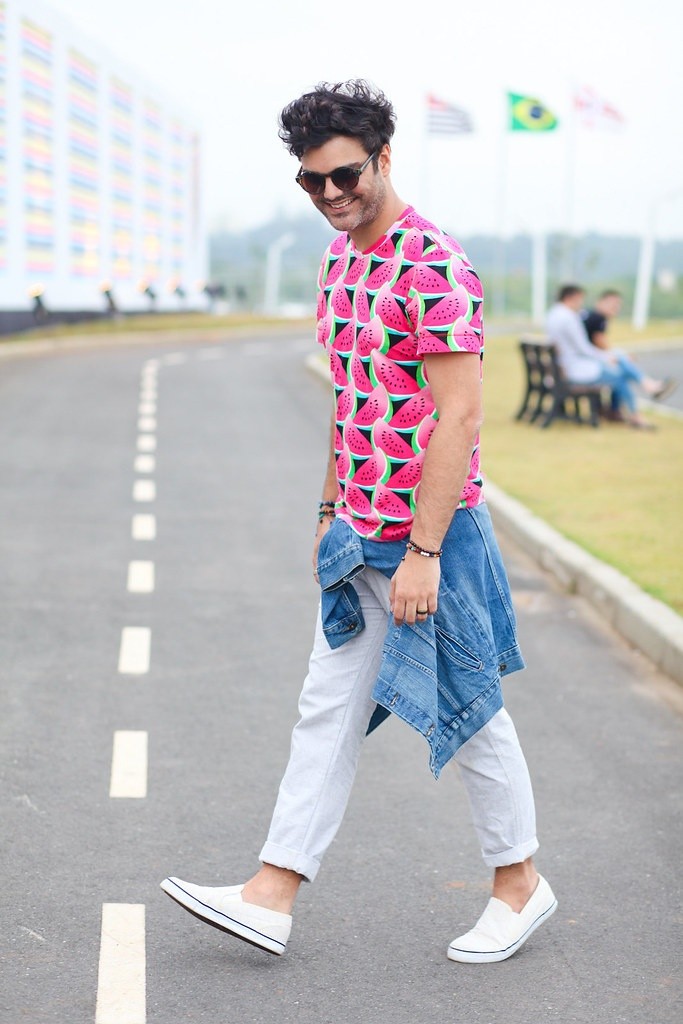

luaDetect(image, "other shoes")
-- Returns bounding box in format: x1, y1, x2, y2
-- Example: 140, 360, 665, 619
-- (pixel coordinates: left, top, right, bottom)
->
447, 873, 558, 964
160, 877, 292, 956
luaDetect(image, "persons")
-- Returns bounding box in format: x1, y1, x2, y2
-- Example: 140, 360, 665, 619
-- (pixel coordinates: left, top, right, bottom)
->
157, 91, 561, 961
550, 285, 654, 430
580, 290, 625, 422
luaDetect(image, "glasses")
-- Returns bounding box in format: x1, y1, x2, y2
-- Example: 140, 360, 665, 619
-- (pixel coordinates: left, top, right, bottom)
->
295, 148, 376, 195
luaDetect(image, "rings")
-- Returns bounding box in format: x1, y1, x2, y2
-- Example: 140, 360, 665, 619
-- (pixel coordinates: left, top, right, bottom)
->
417, 611, 427, 614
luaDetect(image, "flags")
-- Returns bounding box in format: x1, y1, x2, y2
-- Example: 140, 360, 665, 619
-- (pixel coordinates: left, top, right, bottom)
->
509, 93, 557, 132
426, 96, 472, 134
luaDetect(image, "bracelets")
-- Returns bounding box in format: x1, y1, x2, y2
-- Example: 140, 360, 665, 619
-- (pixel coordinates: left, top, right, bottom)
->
318, 500, 336, 524
402, 539, 442, 562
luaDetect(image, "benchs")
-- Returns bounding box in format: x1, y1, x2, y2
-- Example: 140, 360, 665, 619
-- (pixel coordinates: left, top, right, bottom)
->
515, 341, 601, 428
